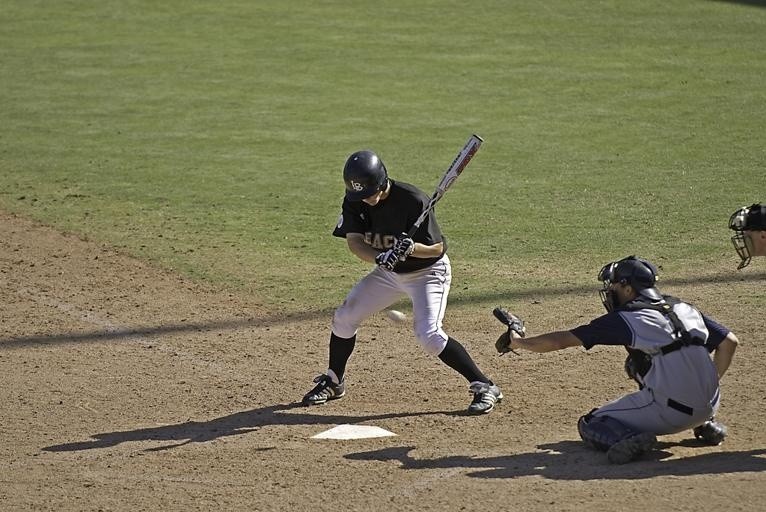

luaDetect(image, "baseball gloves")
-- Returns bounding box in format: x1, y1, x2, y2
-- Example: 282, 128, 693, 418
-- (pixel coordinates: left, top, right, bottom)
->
493, 307, 524, 353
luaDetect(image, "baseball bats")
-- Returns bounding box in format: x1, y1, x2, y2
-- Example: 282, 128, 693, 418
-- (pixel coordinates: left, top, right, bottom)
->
408, 133, 485, 236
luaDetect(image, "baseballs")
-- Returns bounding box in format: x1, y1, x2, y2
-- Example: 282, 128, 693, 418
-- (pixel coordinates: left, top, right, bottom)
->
388, 310, 405, 323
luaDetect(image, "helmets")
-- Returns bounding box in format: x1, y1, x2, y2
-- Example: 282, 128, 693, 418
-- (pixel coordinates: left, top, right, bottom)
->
730, 203, 766, 231
342, 149, 388, 202
616, 258, 663, 303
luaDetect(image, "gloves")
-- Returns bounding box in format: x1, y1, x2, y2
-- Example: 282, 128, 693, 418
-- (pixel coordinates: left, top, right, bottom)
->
394, 232, 415, 256
375, 249, 399, 271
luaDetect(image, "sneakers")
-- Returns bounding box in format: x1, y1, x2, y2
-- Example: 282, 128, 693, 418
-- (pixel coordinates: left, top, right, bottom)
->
696, 420, 727, 446
605, 429, 657, 465
302, 373, 346, 405
467, 382, 504, 415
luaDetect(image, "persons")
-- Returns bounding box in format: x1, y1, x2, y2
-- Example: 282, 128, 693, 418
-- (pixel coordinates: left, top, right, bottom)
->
727, 203, 766, 270
492, 255, 741, 463
302, 148, 504, 416
